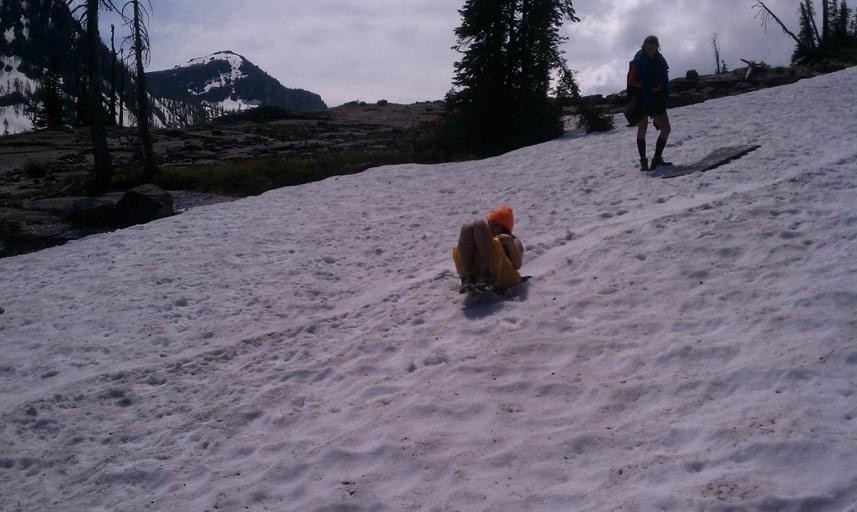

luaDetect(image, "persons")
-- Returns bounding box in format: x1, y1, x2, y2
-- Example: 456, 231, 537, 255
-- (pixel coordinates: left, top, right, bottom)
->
455, 207, 525, 293
625, 35, 673, 170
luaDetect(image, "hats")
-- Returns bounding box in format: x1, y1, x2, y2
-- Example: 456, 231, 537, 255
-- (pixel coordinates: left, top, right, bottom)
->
488, 208, 513, 233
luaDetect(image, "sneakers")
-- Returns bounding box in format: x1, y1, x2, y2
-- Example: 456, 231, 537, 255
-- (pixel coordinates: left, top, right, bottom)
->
640, 158, 650, 171
651, 159, 672, 170
459, 271, 495, 294
